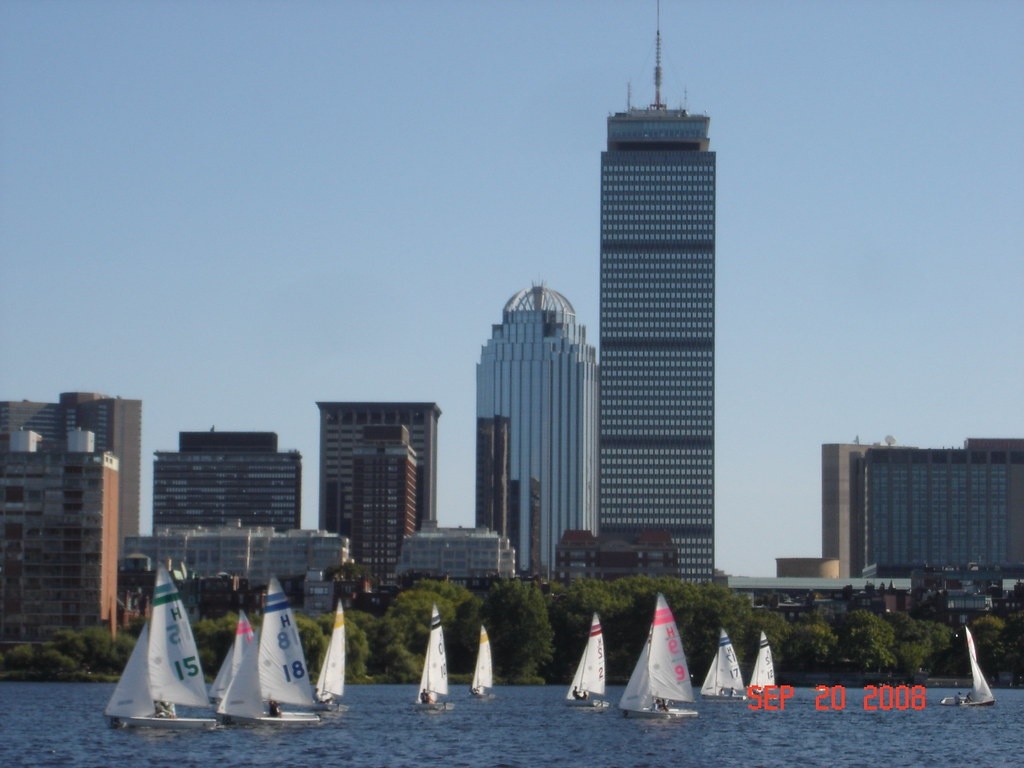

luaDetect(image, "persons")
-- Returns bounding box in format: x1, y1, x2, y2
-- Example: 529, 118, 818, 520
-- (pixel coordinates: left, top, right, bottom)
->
154, 700, 173, 719
954, 690, 963, 704
650, 698, 670, 713
420, 688, 434, 705
572, 686, 589, 701
269, 699, 283, 719
728, 687, 736, 697
312, 688, 333, 705
718, 687, 725, 696
965, 690, 973, 703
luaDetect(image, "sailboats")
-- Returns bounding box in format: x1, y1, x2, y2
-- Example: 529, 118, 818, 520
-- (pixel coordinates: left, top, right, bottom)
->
306, 599, 351, 715
209, 606, 255, 706
698, 627, 750, 703
559, 611, 612, 711
940, 624, 998, 709
100, 562, 218, 732
213, 574, 322, 729
746, 630, 778, 695
465, 623, 497, 700
613, 591, 701, 720
408, 603, 455, 714
